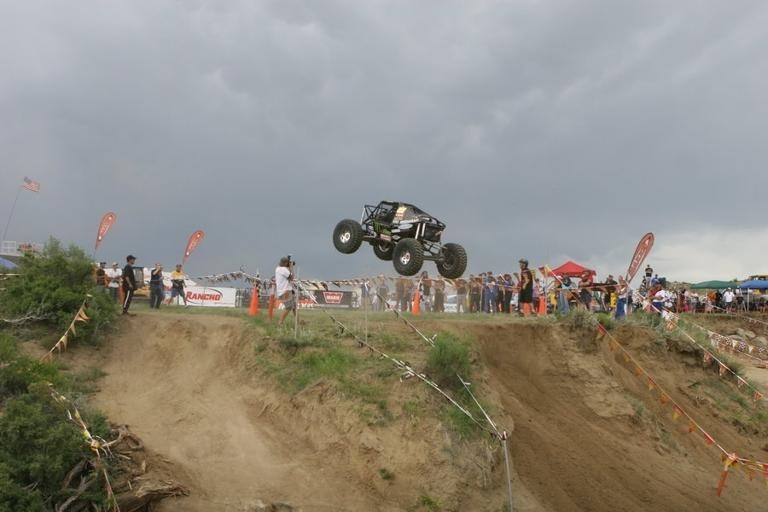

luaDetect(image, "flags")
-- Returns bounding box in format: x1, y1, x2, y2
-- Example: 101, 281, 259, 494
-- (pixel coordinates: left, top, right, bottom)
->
625, 232, 654, 282
23, 177, 40, 192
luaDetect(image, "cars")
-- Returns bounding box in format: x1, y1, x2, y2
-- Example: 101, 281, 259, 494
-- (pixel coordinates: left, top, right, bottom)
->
134, 284, 151, 297
510, 292, 518, 311
693, 275, 767, 314
383, 290, 463, 314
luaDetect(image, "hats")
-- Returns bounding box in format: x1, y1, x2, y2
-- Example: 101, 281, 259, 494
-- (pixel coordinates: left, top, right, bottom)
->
127, 256, 136, 261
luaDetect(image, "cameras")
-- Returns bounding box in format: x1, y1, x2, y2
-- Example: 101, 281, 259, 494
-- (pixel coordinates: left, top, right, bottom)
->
287, 255, 295, 267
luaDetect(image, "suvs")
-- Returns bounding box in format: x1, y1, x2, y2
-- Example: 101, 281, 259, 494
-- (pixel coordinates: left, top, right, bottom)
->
330, 198, 470, 281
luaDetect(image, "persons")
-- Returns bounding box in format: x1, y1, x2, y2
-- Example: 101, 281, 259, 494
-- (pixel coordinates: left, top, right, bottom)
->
275, 257, 310, 326
166, 264, 190, 308
96, 261, 107, 286
360, 259, 768, 317
107, 262, 123, 301
122, 255, 137, 316
149, 263, 164, 310
